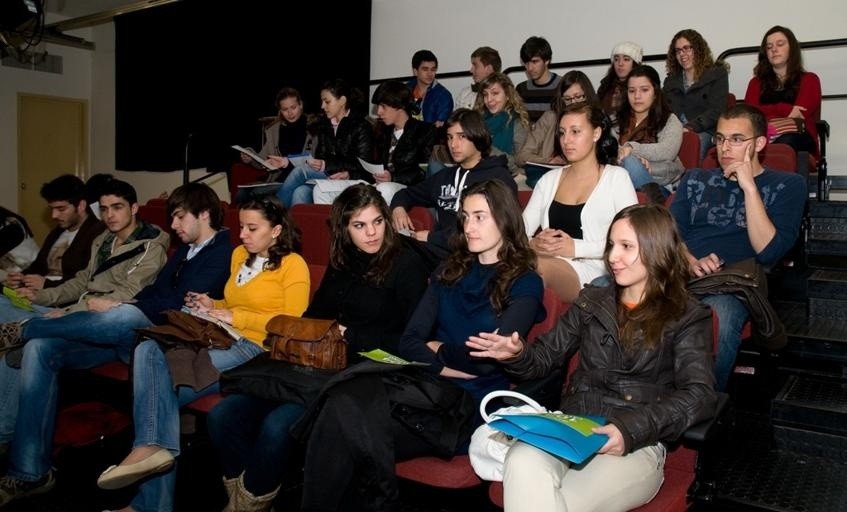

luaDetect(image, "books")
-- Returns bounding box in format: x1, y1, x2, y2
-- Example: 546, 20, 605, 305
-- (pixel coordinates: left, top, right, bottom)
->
179, 304, 240, 341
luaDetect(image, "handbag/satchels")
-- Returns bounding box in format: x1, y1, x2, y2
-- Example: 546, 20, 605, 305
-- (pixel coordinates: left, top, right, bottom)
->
767, 117, 806, 144
218, 350, 338, 406
264, 313, 347, 372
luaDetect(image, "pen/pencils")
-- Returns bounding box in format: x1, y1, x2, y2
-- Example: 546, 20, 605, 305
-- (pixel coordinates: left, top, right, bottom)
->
190, 292, 209, 301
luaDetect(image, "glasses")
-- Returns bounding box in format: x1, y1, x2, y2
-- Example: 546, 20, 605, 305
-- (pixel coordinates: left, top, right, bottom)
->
672, 46, 694, 55
710, 134, 762, 147
561, 94, 587, 104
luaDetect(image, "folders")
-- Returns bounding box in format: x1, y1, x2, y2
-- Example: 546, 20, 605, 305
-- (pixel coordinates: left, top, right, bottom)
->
487, 414, 608, 464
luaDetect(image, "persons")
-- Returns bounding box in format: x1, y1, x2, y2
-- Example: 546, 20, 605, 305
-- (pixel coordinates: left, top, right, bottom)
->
222, 177, 547, 512
96, 195, 310, 512
205, 182, 432, 511
238, 25, 821, 303
668, 104, 807, 392
1, 175, 233, 511
465, 202, 717, 512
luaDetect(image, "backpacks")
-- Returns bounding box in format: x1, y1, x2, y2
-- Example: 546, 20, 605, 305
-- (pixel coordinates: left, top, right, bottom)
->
468, 389, 565, 484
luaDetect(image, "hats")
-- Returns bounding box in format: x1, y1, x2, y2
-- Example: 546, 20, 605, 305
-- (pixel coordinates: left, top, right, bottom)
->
611, 41, 644, 68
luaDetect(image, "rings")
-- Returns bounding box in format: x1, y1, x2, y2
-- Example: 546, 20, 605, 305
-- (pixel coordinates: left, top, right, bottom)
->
486, 344, 495, 350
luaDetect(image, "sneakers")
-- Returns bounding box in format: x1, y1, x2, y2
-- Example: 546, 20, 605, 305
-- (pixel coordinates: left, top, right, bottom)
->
96, 447, 175, 490
0, 318, 31, 352
0, 466, 57, 512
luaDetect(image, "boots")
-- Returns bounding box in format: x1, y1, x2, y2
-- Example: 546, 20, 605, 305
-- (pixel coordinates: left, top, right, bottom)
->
221, 474, 240, 500
223, 470, 285, 512
